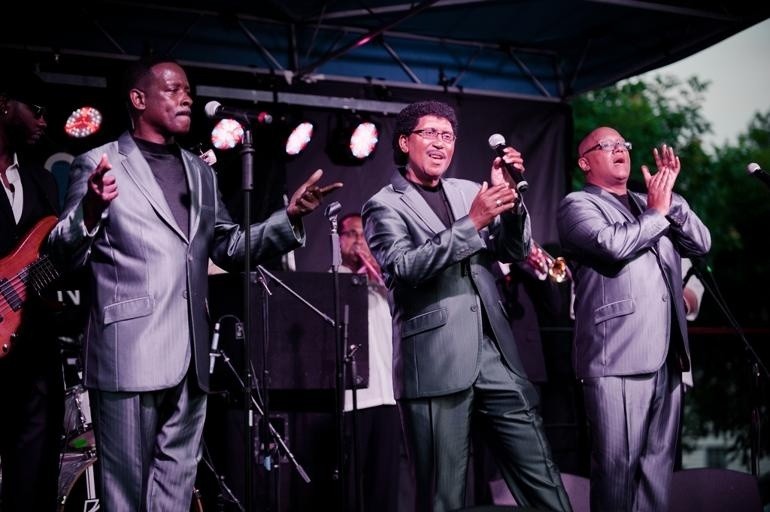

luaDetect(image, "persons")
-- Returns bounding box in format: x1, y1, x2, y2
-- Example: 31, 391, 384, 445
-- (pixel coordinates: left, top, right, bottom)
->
48, 55, 344, 512
558, 125, 712, 512
3, 69, 92, 511
361, 101, 574, 512
675, 257, 706, 472
323, 212, 411, 511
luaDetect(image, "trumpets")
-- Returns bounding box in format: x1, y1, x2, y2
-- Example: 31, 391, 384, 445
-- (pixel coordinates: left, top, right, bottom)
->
532, 243, 568, 284
358, 244, 400, 298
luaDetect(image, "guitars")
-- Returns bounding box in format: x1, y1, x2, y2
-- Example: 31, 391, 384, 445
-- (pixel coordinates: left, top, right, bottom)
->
0, 148, 219, 387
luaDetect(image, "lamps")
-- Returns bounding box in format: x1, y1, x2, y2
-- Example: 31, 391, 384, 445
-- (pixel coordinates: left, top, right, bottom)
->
270, 65, 315, 157
325, 105, 380, 167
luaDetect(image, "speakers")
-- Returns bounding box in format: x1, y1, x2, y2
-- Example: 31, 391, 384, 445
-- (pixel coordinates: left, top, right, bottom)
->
208, 272, 369, 411
666, 465, 764, 512
205, 413, 343, 510
344, 404, 418, 511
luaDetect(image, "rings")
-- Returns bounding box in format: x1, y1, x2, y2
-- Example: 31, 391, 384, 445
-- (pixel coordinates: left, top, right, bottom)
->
495, 199, 504, 208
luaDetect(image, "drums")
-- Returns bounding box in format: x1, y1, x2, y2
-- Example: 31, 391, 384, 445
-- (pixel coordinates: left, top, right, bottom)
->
60, 447, 202, 511
65, 385, 108, 457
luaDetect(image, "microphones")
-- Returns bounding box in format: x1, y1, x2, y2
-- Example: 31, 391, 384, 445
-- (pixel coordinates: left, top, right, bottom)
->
204, 99, 273, 125
747, 162, 769, 189
488, 132, 530, 193
210, 322, 220, 374
